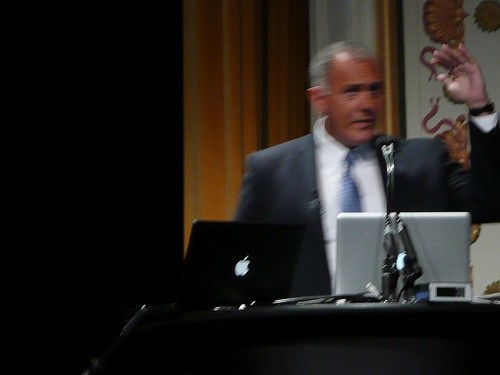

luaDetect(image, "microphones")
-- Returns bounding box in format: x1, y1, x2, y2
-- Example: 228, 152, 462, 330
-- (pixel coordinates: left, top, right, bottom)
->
389, 137, 400, 212
376, 136, 391, 214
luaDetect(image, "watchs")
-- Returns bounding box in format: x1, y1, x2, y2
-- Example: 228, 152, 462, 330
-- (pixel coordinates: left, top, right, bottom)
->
468, 98, 495, 116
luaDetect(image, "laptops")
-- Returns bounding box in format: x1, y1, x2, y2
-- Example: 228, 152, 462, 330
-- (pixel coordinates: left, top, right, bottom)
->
325, 212, 471, 303
141, 219, 306, 313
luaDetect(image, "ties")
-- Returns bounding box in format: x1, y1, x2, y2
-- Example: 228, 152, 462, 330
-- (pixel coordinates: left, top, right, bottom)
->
336, 151, 361, 212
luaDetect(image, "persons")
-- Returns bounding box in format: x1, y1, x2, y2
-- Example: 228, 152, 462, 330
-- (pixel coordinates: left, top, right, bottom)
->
235, 39, 500, 305
